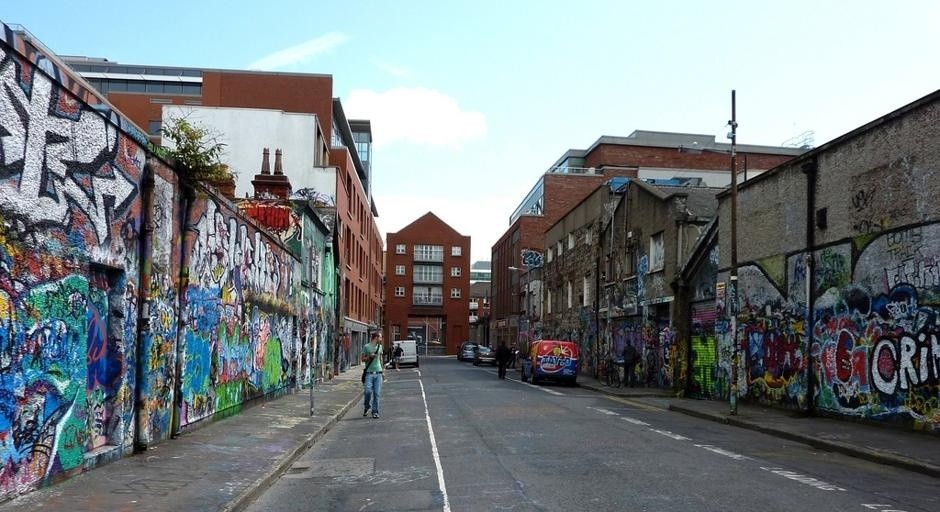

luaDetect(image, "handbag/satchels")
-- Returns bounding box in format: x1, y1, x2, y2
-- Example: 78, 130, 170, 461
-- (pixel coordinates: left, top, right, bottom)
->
361, 369, 367, 383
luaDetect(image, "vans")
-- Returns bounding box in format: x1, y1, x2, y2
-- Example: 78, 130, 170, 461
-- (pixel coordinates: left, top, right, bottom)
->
521, 339, 579, 388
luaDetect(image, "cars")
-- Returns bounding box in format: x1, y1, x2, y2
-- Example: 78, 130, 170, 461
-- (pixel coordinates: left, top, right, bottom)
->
457, 341, 498, 367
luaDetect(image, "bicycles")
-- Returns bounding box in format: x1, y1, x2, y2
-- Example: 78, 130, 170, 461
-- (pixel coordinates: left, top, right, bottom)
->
596, 356, 621, 388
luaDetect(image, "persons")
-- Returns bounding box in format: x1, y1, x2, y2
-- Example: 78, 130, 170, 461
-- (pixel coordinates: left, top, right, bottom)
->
362, 331, 387, 419
392, 343, 404, 373
507, 343, 519, 368
622, 339, 640, 388
496, 340, 511, 380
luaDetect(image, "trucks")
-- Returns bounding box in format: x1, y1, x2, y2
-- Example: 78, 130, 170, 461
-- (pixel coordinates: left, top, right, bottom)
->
392, 340, 419, 369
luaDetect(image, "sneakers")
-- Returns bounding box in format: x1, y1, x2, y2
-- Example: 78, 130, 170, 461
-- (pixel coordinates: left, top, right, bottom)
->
396, 366, 400, 372
363, 404, 372, 416
371, 412, 380, 419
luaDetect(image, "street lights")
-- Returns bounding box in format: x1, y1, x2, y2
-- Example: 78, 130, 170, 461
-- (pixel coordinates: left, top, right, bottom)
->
508, 265, 531, 344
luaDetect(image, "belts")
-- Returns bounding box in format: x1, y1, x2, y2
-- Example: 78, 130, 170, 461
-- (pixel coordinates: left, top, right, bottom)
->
366, 371, 382, 374
